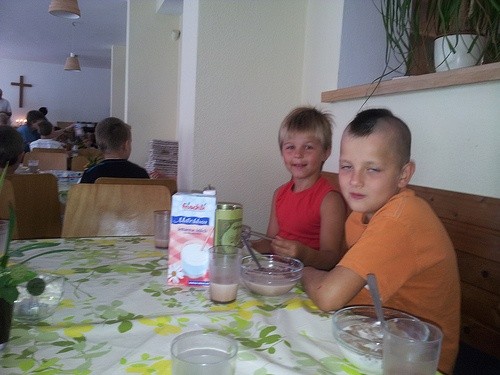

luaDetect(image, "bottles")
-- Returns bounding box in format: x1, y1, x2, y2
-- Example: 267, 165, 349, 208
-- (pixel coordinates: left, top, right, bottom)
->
216, 203, 243, 258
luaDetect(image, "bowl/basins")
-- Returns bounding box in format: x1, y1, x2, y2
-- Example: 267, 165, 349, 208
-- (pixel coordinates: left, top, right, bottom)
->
330, 305, 430, 375
240, 254, 304, 296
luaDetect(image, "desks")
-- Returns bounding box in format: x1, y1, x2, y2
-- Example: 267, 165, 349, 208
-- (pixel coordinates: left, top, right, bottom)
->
15, 169, 86, 205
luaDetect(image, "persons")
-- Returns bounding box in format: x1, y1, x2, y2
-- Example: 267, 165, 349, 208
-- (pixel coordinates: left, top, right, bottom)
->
0, 125, 24, 220
0, 89, 12, 125
81, 117, 148, 184
291, 109, 461, 375
247, 107, 349, 271
17, 107, 98, 152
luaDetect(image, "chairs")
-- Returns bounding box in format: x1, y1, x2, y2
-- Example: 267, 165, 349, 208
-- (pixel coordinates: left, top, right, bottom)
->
28, 152, 68, 170
31, 148, 65, 153
70, 149, 102, 171
97, 177, 179, 193
23, 152, 29, 166
6, 173, 62, 238
0, 178, 20, 240
61, 181, 171, 238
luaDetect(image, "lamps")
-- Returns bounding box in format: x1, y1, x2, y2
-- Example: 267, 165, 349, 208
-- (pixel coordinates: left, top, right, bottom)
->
63, 22, 82, 71
47, 0, 81, 19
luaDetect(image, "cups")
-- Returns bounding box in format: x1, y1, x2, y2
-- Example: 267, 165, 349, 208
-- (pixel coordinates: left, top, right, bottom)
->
208, 245, 242, 303
382, 318, 444, 375
153, 210, 170, 248
27, 159, 39, 173
0, 219, 10, 256
171, 329, 238, 375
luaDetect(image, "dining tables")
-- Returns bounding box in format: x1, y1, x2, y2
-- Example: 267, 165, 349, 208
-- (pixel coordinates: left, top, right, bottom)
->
0, 237, 442, 375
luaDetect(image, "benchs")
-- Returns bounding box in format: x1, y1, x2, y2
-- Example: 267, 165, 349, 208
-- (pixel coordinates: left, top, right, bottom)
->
320, 171, 500, 375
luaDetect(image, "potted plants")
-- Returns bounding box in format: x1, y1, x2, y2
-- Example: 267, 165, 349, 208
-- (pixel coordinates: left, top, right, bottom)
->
356, 0, 500, 117
